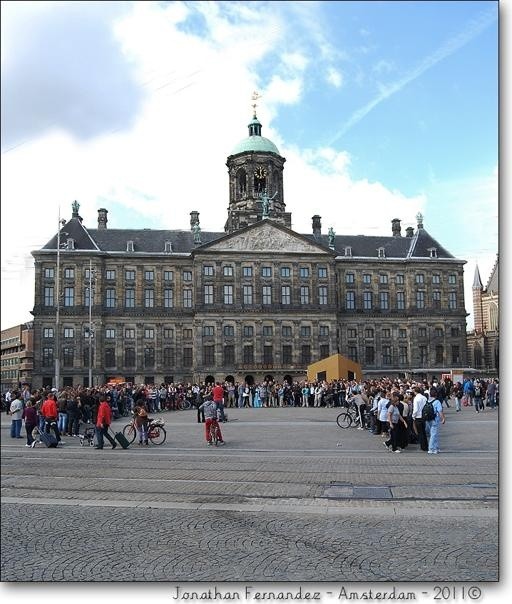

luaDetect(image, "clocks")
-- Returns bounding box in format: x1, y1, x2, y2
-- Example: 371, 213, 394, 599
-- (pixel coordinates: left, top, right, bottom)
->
254, 165, 268, 178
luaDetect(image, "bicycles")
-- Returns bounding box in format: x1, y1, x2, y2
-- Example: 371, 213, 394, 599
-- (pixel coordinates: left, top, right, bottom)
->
177, 394, 192, 410
336, 399, 374, 432
122, 408, 167, 446
209, 415, 219, 444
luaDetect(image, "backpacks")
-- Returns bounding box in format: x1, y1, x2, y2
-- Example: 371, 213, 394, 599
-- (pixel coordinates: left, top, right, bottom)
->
422, 400, 436, 420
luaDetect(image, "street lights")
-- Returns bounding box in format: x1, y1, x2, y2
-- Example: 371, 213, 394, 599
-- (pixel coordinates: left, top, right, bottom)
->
52, 203, 71, 388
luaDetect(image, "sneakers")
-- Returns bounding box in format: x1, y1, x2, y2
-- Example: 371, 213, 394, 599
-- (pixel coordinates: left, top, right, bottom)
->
383, 441, 388, 450
392, 450, 401, 453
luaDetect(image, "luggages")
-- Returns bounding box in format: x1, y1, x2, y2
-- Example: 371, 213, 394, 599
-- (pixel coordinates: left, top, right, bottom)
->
107, 425, 130, 449
34, 426, 58, 448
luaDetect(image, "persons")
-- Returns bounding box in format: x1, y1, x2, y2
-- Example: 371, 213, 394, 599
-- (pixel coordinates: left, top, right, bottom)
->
6, 377, 498, 455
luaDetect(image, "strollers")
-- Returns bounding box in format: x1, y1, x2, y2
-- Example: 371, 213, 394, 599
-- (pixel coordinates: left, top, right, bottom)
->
78, 421, 97, 447
